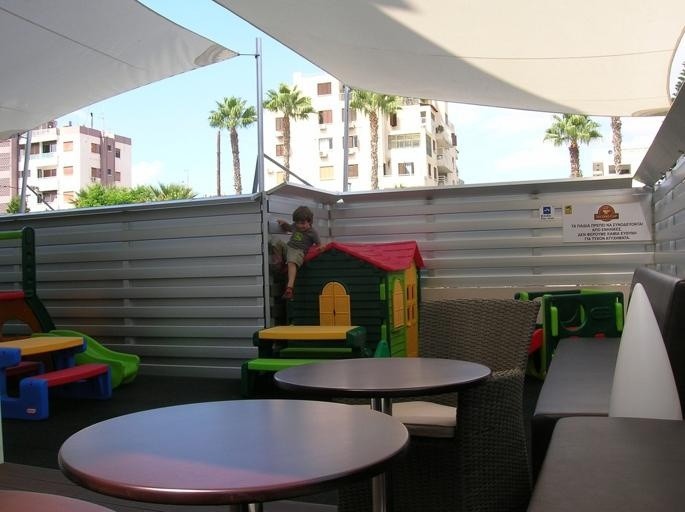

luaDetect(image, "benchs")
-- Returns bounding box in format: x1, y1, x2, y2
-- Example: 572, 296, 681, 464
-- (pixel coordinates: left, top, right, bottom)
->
530, 269, 684, 472
519, 414, 685, 511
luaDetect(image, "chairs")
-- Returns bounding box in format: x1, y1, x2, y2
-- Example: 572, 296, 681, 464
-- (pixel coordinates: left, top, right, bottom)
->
331, 299, 541, 510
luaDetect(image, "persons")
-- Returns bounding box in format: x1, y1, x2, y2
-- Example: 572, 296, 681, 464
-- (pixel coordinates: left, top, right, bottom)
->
269, 205, 322, 299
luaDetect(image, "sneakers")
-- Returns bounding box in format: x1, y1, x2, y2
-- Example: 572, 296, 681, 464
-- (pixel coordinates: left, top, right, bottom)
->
281, 287, 294, 299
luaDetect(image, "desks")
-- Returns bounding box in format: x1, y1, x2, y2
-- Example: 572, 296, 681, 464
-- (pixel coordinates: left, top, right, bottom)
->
57, 398, 410, 511
0, 491, 118, 512
273, 357, 491, 512
